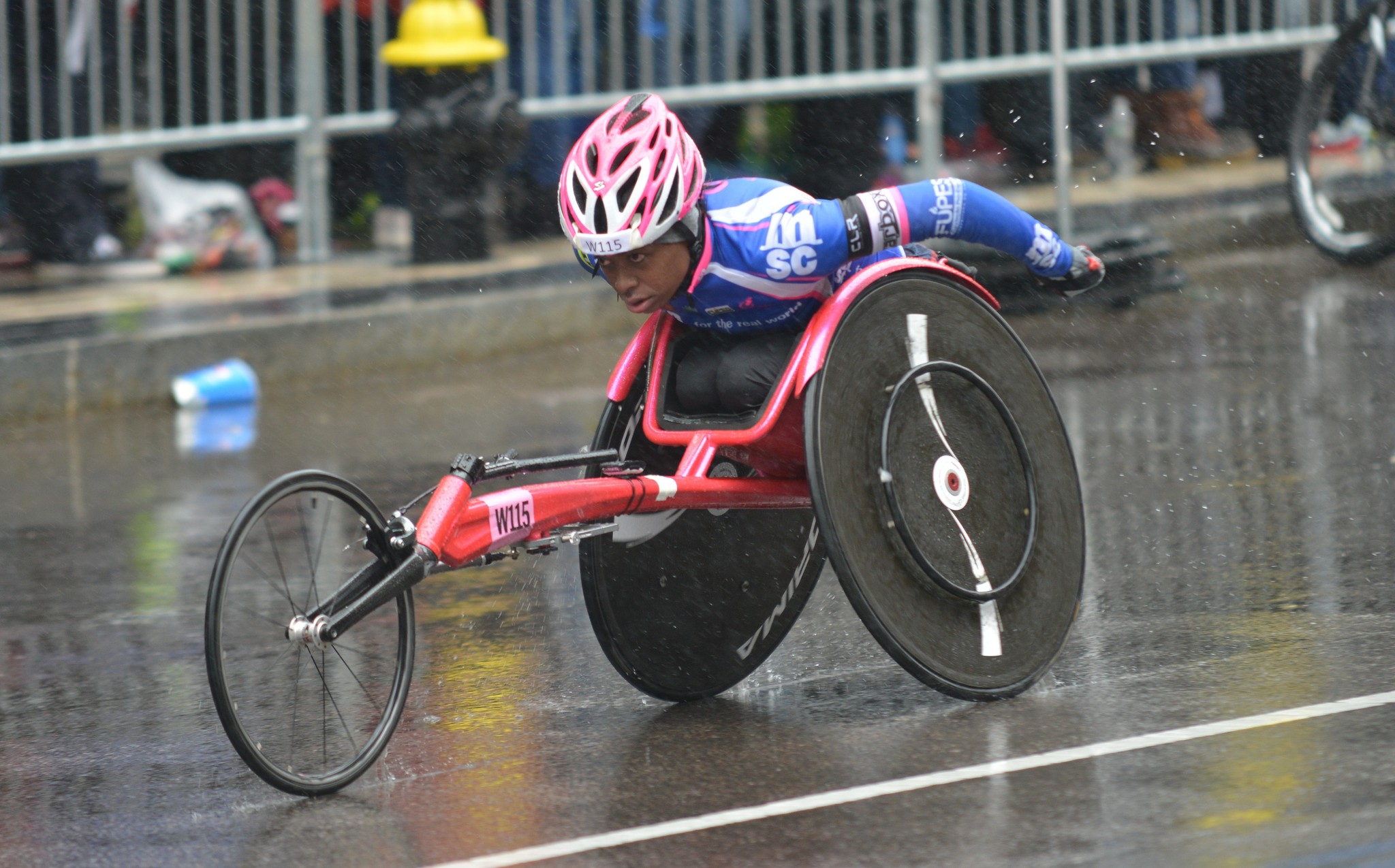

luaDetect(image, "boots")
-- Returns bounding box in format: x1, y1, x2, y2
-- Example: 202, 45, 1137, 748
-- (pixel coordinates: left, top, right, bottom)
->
1135, 86, 1223, 161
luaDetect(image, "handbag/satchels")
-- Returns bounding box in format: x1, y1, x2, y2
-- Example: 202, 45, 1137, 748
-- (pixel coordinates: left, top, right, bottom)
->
131, 154, 272, 276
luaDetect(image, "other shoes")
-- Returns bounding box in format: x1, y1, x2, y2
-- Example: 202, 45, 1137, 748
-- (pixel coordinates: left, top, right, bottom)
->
0, 224, 68, 289
1309, 111, 1374, 152
966, 125, 1009, 176
880, 131, 979, 183
35, 229, 165, 279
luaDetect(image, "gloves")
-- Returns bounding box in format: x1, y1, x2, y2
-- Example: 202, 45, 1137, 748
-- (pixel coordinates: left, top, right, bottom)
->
1023, 244, 1105, 300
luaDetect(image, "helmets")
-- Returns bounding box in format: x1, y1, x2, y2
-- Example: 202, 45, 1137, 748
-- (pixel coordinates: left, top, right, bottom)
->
555, 92, 707, 257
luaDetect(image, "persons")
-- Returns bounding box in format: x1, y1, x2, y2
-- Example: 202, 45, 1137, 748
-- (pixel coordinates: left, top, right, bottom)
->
2, 0, 1394, 269
554, 92, 1107, 419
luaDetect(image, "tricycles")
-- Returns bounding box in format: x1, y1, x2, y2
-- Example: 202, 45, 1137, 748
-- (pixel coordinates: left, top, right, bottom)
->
205, 255, 1087, 800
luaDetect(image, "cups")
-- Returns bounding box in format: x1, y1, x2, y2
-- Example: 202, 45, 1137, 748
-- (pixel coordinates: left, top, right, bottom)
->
174, 358, 259, 409
